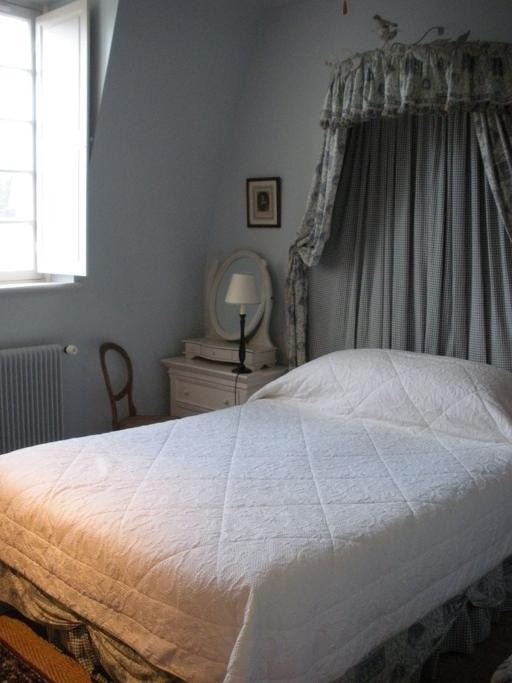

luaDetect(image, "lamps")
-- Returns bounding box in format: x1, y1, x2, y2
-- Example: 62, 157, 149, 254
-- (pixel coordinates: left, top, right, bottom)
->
224, 272, 261, 375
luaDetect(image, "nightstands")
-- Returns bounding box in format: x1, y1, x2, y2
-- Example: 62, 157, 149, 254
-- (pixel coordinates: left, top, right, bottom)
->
162, 357, 287, 417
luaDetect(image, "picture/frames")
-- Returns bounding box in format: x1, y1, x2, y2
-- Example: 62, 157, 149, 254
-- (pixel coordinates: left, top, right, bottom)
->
246, 177, 281, 227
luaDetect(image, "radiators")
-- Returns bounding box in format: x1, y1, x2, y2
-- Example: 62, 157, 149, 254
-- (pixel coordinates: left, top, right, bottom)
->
0, 343, 67, 456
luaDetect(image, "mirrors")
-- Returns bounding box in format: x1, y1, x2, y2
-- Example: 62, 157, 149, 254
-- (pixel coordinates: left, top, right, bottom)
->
209, 250, 268, 341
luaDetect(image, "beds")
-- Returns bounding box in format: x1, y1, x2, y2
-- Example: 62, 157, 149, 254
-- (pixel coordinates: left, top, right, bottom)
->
1, 347, 512, 683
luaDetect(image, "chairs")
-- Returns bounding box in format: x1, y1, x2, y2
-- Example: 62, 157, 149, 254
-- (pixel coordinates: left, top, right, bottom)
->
99, 342, 181, 428
